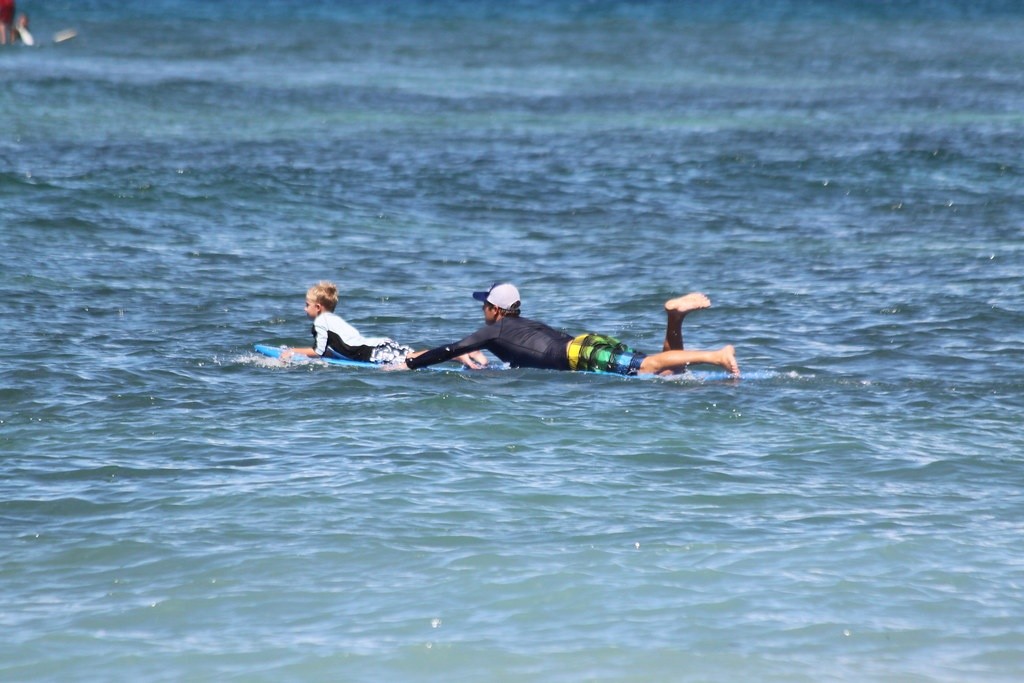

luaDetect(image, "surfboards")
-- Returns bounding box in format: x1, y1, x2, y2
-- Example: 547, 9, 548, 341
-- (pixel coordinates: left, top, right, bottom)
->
252, 341, 779, 388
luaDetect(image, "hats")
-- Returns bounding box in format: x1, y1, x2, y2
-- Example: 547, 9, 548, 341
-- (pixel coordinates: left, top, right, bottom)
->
472, 282, 521, 310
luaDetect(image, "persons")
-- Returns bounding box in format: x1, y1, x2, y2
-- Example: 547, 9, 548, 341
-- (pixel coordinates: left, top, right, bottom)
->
0, 0, 32, 46
285, 282, 489, 370
382, 280, 739, 375
